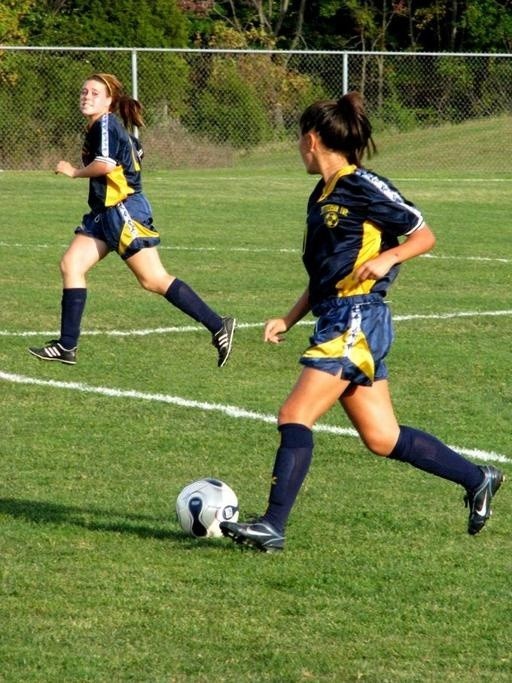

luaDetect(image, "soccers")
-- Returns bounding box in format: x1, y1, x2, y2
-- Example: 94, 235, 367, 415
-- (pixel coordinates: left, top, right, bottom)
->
174, 479, 242, 541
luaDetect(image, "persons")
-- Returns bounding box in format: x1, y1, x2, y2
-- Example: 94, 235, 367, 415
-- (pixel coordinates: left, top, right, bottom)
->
219, 91, 505, 552
28, 73, 236, 368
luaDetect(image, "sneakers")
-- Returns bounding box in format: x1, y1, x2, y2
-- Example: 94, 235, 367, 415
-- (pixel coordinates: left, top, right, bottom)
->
212, 316, 236, 368
465, 465, 503, 536
28, 343, 78, 365
219, 516, 286, 554
